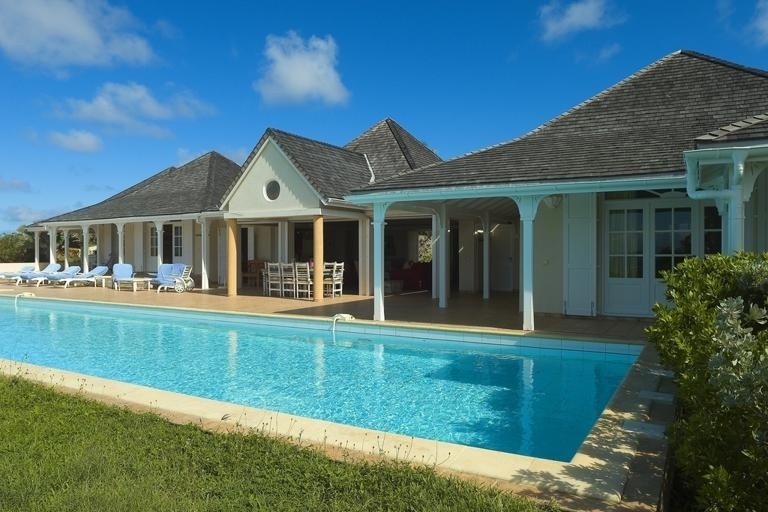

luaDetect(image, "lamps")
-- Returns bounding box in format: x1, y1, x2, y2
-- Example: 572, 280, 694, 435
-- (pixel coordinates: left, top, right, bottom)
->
544, 193, 562, 208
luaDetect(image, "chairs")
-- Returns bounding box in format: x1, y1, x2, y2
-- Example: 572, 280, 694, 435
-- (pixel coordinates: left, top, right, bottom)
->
243, 259, 345, 299
389, 262, 430, 289
0, 263, 195, 292
